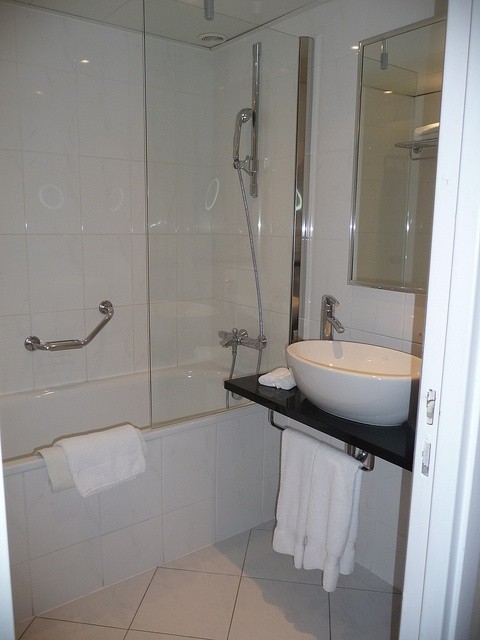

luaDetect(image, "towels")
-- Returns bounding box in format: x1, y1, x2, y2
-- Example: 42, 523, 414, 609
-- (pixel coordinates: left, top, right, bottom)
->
413, 123, 439, 140
36, 422, 150, 499
270, 428, 364, 594
257, 366, 296, 391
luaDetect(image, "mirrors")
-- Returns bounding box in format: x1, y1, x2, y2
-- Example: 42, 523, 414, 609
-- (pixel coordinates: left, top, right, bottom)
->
345, 16, 446, 296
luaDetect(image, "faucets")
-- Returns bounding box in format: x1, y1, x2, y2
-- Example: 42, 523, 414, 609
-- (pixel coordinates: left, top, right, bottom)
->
320, 294, 345, 340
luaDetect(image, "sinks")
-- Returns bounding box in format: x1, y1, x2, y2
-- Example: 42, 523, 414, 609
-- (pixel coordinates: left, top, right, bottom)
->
285, 341, 422, 428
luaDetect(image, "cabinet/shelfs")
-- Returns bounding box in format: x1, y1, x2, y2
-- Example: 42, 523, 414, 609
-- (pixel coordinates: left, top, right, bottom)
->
220, 368, 414, 474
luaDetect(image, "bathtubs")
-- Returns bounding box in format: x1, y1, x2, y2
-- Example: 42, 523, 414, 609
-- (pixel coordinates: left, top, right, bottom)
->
0, 366, 255, 463
